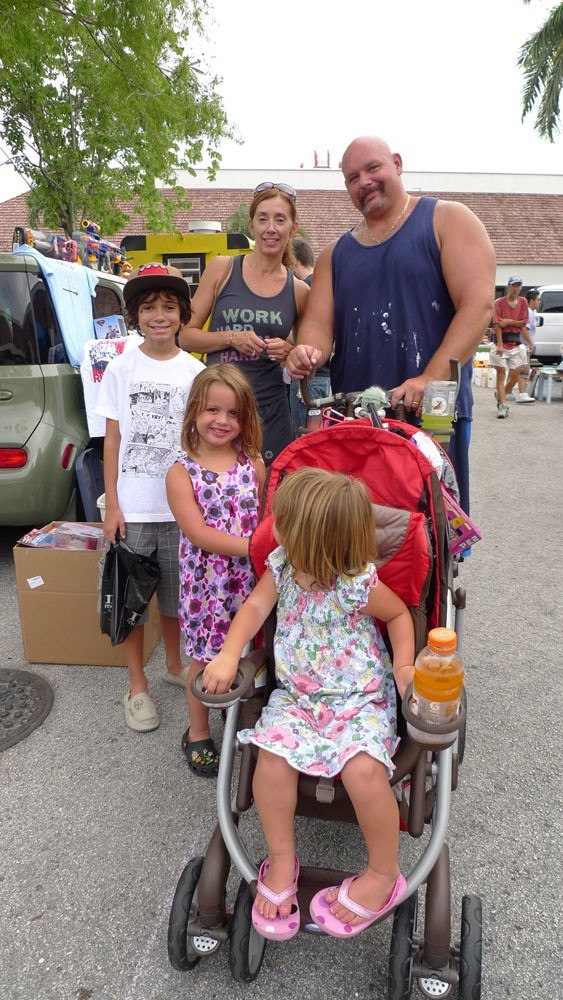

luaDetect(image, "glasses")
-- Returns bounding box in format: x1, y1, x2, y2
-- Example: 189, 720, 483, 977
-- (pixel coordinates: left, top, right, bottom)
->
254, 182, 297, 202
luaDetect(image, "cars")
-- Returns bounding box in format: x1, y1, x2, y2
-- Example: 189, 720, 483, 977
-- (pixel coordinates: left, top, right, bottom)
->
523, 283, 563, 367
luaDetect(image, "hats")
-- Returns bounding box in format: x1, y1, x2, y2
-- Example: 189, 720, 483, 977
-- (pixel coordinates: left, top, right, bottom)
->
123, 262, 190, 300
508, 275, 523, 285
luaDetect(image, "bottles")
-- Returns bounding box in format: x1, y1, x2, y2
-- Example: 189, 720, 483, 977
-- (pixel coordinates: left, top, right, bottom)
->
406, 627, 463, 746
421, 381, 459, 452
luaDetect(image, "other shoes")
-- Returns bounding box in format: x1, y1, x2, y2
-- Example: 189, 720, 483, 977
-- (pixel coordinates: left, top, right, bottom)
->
123, 688, 159, 732
161, 667, 191, 690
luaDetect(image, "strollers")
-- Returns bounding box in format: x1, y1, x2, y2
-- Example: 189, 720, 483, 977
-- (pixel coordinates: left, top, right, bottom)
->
167, 355, 490, 1000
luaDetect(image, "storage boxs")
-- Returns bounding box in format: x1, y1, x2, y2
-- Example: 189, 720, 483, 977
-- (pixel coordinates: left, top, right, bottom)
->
12, 520, 163, 667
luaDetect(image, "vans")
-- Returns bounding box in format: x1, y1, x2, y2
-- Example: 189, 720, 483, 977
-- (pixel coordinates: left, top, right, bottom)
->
0, 221, 135, 538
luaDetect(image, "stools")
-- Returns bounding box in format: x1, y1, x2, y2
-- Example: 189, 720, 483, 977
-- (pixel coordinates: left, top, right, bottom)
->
530, 367, 563, 405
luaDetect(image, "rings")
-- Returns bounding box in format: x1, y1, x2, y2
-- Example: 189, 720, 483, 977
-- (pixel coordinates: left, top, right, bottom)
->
412, 401, 419, 406
271, 358, 277, 361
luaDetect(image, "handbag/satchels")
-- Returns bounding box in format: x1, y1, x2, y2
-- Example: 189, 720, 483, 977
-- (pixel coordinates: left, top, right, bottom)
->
101, 536, 162, 646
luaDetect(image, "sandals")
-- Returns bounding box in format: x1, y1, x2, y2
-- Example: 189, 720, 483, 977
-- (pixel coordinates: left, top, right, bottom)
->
251, 852, 301, 941
310, 870, 408, 939
181, 726, 220, 778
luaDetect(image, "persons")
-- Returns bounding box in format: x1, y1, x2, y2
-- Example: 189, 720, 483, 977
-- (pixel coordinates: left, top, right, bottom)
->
165, 360, 267, 774
181, 135, 541, 557
203, 466, 425, 939
289, 238, 332, 436
492, 276, 528, 419
95, 262, 206, 733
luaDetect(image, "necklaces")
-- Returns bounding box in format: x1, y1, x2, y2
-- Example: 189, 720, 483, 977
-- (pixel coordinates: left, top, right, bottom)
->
364, 195, 411, 243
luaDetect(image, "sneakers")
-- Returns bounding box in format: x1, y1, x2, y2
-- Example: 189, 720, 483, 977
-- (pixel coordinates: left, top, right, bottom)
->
498, 404, 509, 418
506, 392, 518, 401
516, 392, 535, 403
494, 392, 498, 401
